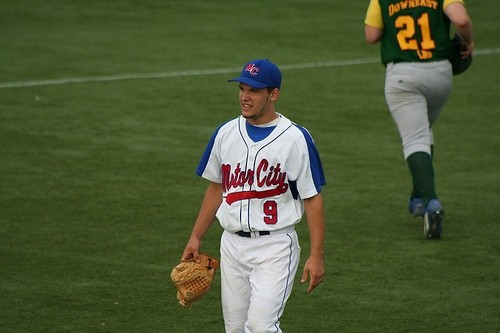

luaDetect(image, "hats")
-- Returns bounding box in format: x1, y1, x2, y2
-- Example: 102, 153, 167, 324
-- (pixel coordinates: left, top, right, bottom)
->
227, 59, 282, 90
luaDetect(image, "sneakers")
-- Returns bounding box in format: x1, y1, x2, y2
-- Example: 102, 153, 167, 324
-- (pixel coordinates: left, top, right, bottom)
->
408, 198, 425, 218
423, 199, 444, 240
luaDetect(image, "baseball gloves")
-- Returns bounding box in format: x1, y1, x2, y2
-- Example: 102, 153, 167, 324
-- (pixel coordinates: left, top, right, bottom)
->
449, 34, 473, 76
172, 254, 217, 307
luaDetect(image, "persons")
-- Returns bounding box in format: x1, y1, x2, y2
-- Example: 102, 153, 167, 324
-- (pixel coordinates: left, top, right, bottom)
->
364, 0, 474, 239
180, 58, 327, 333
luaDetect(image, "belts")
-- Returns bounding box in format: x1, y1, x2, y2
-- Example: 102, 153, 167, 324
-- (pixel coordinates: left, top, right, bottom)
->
235, 230, 269, 237
